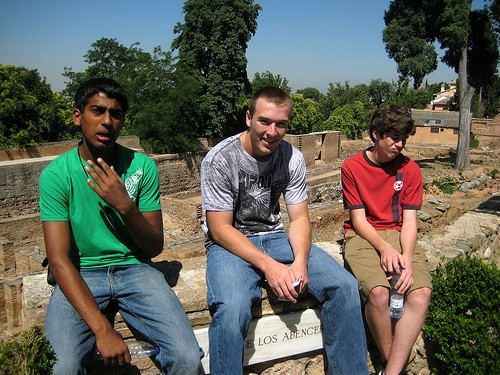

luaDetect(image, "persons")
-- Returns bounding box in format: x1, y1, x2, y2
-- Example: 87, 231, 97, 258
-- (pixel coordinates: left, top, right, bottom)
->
340, 104, 433, 375
199, 87, 369, 375
37, 77, 205, 375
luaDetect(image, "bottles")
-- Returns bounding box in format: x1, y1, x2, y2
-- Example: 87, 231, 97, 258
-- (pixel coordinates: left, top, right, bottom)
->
96, 341, 160, 359
389, 268, 404, 319
